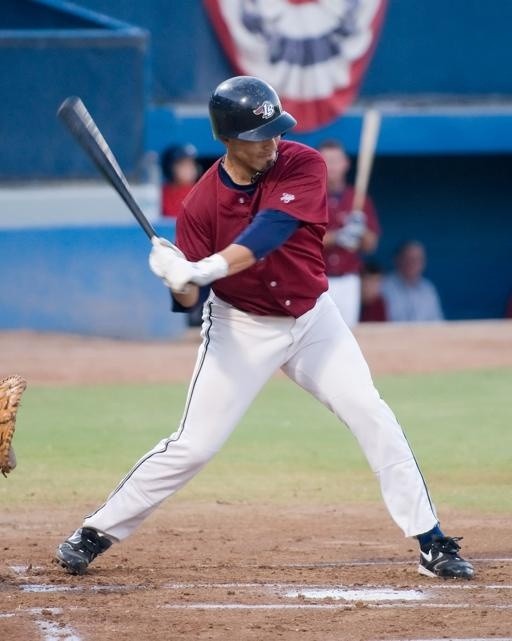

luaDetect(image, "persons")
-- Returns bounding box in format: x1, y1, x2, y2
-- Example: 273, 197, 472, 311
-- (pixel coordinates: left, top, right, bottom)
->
158, 140, 209, 328
53, 73, 476, 582
376, 235, 443, 322
308, 143, 379, 330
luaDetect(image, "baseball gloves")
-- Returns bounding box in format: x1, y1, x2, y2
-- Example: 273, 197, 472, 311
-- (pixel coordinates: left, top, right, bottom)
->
0, 376, 25, 475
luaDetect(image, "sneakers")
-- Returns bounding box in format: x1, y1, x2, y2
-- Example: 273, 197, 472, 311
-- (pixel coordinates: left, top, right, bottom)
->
418, 537, 475, 579
55, 527, 112, 574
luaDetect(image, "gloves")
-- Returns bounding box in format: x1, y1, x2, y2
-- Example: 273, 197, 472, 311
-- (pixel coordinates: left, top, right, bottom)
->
335, 212, 366, 250
148, 236, 228, 290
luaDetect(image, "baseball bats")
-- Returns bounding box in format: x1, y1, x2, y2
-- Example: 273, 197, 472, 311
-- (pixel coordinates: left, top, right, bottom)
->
351, 111, 383, 215
56, 96, 156, 240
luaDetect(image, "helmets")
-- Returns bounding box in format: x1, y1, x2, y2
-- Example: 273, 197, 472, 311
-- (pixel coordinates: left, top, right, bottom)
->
209, 77, 297, 141
